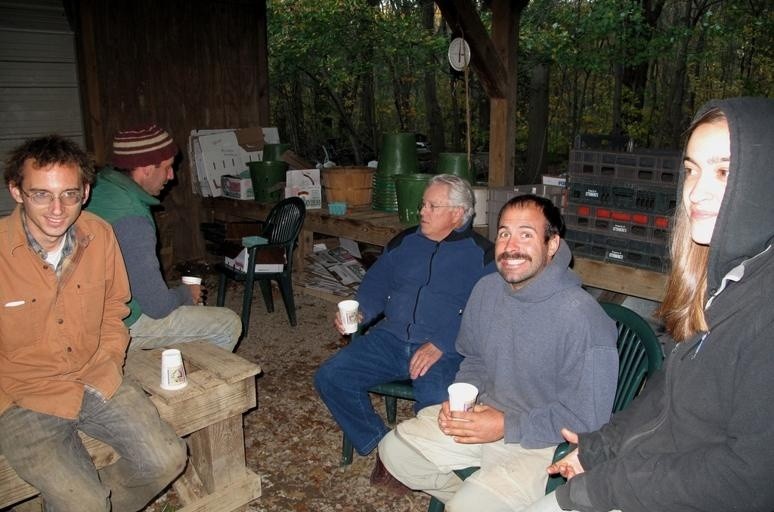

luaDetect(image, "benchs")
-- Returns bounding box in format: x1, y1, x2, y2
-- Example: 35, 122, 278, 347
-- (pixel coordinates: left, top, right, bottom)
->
0, 335, 264, 510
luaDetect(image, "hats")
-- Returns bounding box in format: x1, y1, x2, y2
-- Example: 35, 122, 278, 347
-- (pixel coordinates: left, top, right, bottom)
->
111, 121, 180, 168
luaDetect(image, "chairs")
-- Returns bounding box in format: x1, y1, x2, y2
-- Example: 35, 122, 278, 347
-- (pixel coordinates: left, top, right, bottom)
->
422, 303, 662, 510
210, 195, 307, 336
338, 311, 422, 462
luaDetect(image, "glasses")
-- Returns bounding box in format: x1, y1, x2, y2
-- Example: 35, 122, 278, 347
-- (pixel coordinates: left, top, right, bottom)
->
19, 188, 83, 205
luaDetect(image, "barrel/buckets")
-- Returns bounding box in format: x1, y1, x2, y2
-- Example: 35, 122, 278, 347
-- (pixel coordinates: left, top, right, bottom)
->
324, 166, 374, 211
370, 132, 474, 228
246, 144, 293, 203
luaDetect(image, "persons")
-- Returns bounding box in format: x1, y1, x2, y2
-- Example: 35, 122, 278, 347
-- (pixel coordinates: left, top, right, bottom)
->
375, 195, 622, 512
82, 120, 244, 353
0, 134, 190, 512
546, 94, 774, 512
312, 170, 498, 494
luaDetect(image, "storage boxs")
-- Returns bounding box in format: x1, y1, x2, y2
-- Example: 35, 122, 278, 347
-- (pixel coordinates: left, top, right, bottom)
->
222, 237, 287, 275
483, 183, 572, 247
186, 125, 321, 211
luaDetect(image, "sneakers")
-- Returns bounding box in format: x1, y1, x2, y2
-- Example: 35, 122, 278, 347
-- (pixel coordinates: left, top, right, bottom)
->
370, 452, 412, 500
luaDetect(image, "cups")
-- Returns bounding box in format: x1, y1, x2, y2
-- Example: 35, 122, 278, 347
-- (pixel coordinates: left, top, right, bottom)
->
338, 299, 359, 333
181, 275, 204, 286
446, 380, 480, 422
159, 348, 190, 391
